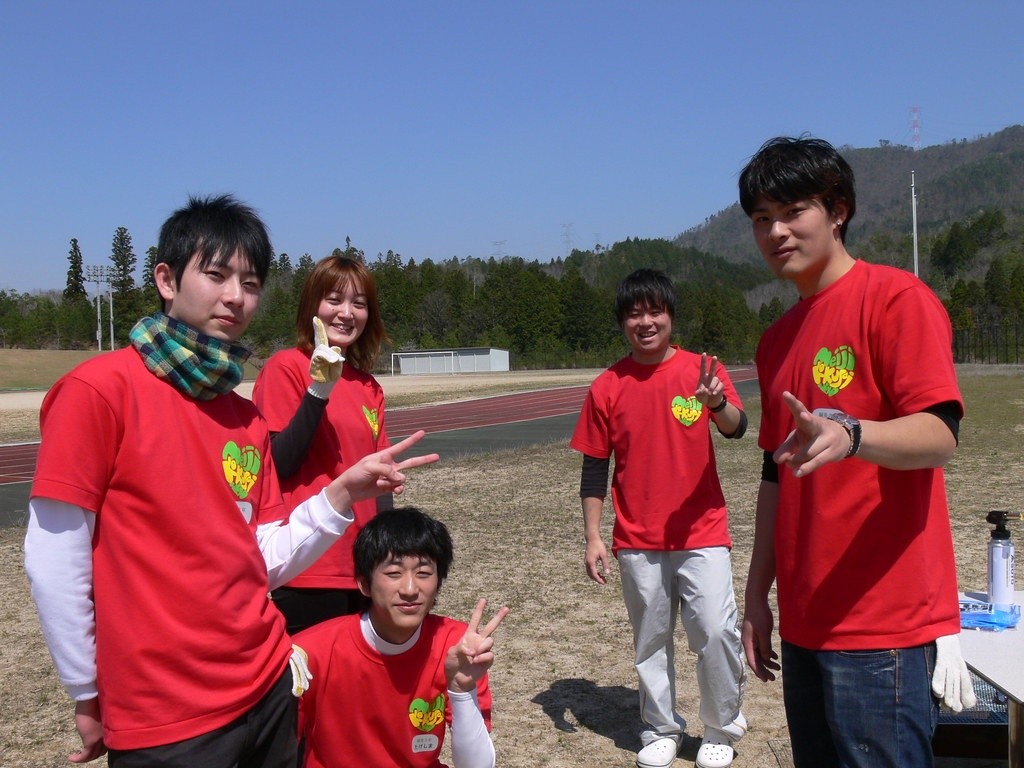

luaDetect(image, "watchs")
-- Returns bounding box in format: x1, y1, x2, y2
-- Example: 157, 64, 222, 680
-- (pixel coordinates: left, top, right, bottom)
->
827, 413, 862, 459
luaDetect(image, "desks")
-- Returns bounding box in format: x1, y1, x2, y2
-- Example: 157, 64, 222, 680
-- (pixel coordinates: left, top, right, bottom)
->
955, 584, 1024, 768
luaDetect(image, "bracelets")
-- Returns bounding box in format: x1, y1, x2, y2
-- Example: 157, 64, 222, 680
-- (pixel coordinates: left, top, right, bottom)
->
710, 394, 727, 413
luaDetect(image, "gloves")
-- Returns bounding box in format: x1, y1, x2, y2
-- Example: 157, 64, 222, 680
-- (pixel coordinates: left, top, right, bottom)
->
307, 316, 346, 400
287, 643, 313, 698
932, 635, 976, 715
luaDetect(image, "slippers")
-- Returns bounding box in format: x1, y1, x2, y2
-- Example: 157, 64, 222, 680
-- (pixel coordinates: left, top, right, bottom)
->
695, 725, 733, 768
637, 734, 683, 768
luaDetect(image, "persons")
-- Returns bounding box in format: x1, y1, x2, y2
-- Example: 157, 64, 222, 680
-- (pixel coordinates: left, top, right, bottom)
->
569, 268, 748, 768
251, 255, 395, 768
739, 131, 966, 768
25, 191, 440, 768
290, 507, 510, 768
